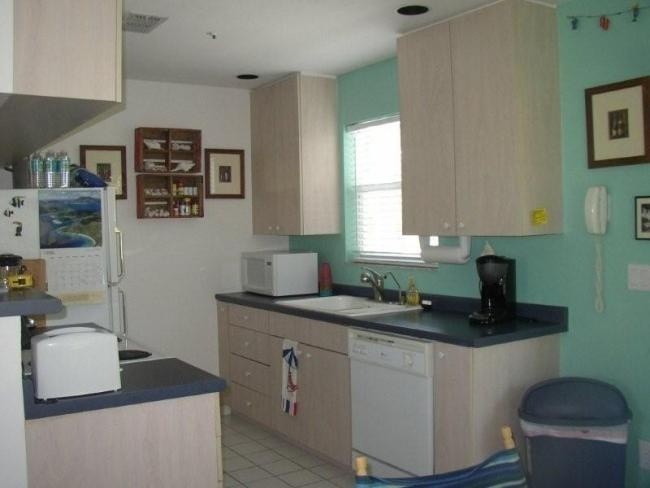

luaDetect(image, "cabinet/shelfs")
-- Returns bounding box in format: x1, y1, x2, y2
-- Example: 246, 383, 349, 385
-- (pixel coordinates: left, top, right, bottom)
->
394, 0, 566, 239
0, 1, 124, 171
250, 70, 339, 238
203, 146, 245, 201
228, 308, 269, 430
268, 313, 352, 470
134, 173, 205, 221
25, 392, 224, 488
133, 125, 203, 175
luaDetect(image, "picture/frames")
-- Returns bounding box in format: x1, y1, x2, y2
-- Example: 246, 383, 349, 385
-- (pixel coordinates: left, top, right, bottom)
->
634, 193, 649, 242
77, 143, 129, 202
583, 74, 650, 171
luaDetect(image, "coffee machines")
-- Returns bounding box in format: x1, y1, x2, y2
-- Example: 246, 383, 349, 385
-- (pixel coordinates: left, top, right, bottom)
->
466, 254, 525, 336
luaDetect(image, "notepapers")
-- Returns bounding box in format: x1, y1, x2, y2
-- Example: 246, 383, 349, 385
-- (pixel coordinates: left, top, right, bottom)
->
531, 209, 548, 226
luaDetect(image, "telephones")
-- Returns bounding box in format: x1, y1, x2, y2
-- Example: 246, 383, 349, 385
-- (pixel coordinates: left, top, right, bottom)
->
584, 185, 608, 314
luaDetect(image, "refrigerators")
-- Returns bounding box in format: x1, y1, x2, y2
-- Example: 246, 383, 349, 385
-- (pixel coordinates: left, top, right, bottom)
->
1, 187, 130, 337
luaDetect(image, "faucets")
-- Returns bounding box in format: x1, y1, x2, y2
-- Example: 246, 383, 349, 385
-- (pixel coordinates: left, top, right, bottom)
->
360, 266, 385, 303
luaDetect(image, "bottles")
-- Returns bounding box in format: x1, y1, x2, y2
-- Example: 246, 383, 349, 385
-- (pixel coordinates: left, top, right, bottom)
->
178, 183, 182, 196
180, 205, 185, 216
192, 204, 198, 216
406, 270, 420, 307
44, 150, 58, 188
182, 178, 198, 197
58, 150, 70, 188
173, 200, 180, 216
184, 199, 192, 216
31, 151, 44, 188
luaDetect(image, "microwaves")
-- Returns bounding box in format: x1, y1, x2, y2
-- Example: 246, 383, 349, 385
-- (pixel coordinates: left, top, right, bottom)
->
241, 250, 319, 296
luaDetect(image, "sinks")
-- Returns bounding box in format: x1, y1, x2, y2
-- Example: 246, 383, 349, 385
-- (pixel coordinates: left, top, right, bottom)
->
275, 295, 424, 317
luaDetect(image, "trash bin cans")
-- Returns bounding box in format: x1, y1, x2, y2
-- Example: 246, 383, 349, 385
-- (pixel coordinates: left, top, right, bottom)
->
518, 377, 632, 488
